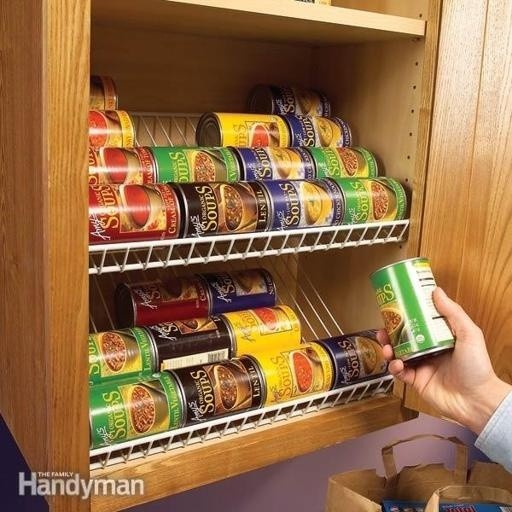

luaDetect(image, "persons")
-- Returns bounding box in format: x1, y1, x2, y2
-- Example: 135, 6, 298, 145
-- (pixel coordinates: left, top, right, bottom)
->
374, 285, 512, 476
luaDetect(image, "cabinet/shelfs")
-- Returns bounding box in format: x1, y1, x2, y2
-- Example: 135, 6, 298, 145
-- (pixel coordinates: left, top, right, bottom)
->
1, 1, 511, 512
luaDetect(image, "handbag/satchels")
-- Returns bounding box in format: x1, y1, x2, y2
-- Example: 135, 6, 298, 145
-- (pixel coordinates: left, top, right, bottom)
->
324, 433, 512, 512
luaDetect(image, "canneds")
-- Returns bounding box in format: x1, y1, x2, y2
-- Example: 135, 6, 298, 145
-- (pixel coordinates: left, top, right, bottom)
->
88, 147, 156, 185
219, 305, 301, 357
148, 143, 240, 186
84, 327, 155, 388
301, 144, 377, 182
166, 180, 270, 238
163, 357, 264, 429
326, 175, 407, 225
246, 82, 333, 117
115, 275, 208, 331
256, 177, 343, 229
88, 371, 185, 451
87, 184, 181, 246
194, 111, 289, 149
89, 74, 118, 110
230, 145, 314, 182
283, 114, 353, 147
87, 110, 134, 148
315, 329, 396, 393
368, 256, 457, 365
146, 313, 231, 373
239, 341, 336, 407
202, 269, 275, 315
